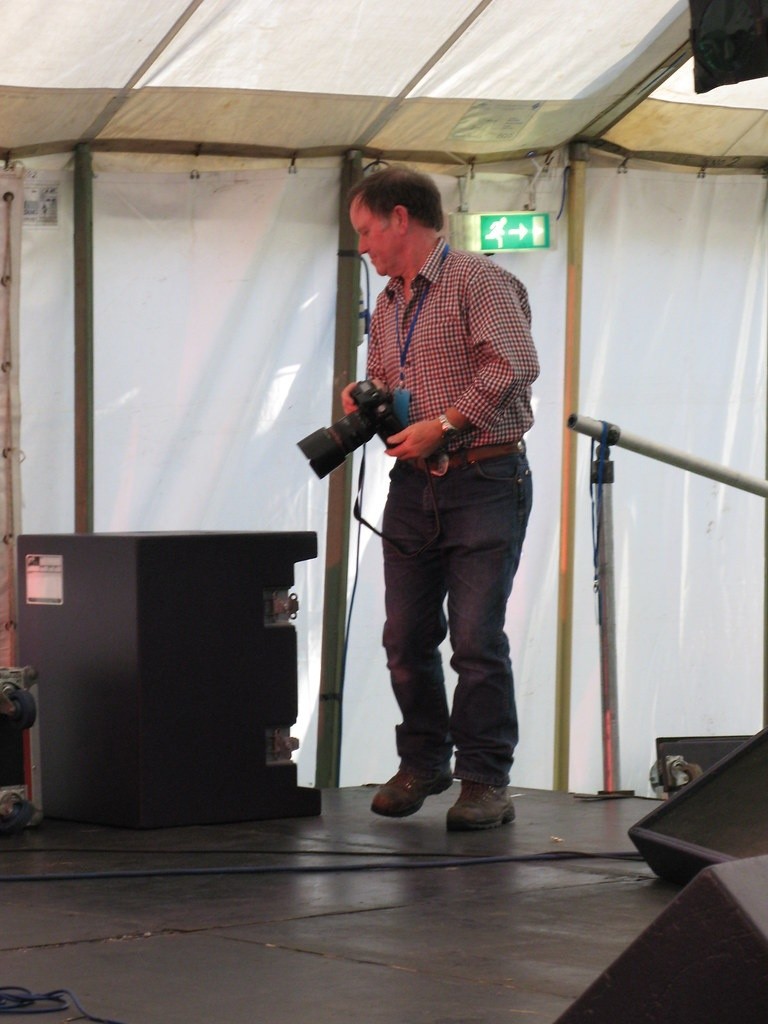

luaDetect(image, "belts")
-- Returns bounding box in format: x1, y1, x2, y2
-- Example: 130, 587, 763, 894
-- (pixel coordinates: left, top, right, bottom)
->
403, 442, 527, 476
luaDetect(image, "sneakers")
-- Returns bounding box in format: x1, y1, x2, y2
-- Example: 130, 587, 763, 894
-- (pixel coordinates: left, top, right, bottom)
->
370, 757, 455, 819
446, 781, 517, 829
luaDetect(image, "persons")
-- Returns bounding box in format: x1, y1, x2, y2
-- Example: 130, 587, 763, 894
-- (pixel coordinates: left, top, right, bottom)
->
343, 166, 540, 830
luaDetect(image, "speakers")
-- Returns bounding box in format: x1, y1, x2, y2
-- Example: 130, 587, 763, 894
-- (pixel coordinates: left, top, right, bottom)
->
550, 854, 768, 1024
628, 727, 768, 889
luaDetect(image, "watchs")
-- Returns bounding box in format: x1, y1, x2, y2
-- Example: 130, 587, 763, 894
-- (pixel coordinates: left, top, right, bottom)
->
438, 414, 458, 438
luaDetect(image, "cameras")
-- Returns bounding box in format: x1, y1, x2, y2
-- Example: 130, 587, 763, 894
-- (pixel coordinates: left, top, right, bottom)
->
296, 379, 406, 478
